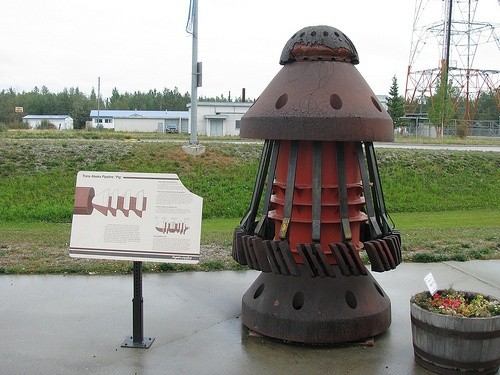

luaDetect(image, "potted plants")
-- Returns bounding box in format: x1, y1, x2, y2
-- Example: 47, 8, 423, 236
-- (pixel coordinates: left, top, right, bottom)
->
409, 290, 500, 375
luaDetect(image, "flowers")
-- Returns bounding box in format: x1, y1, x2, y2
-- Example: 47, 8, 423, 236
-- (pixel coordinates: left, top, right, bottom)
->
409, 288, 500, 318
